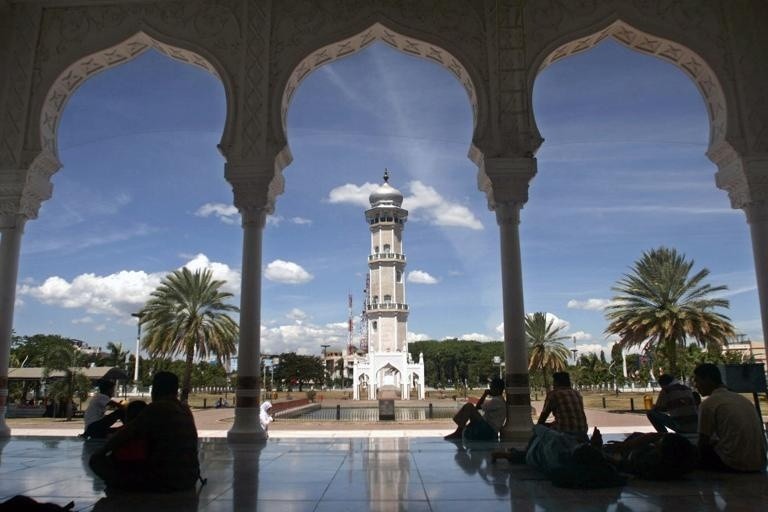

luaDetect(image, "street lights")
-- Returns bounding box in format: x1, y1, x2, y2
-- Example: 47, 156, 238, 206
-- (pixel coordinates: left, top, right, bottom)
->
571, 350, 578, 362
130, 312, 149, 392
321, 344, 331, 358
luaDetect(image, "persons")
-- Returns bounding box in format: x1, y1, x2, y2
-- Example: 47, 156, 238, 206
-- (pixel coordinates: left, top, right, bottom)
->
214, 396, 228, 408
693, 363, 768, 474
82, 378, 124, 436
260, 401, 273, 439
504, 423, 620, 489
646, 374, 700, 434
443, 379, 508, 441
7, 387, 54, 409
88, 370, 201, 492
534, 371, 588, 434
603, 431, 689, 480
